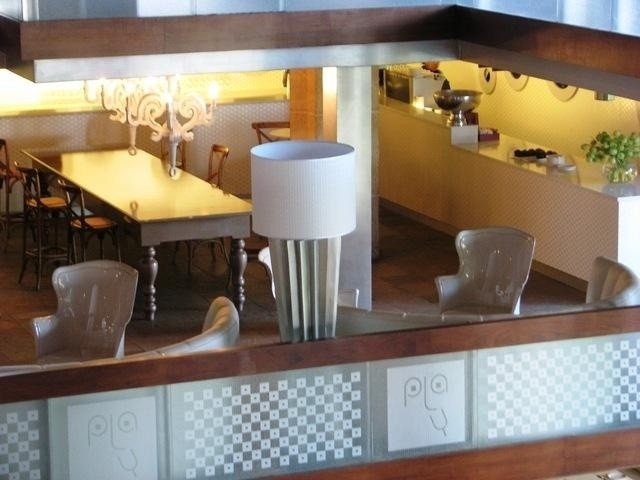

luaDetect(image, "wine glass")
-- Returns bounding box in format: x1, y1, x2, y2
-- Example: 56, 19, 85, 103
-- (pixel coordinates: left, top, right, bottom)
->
433, 89, 483, 126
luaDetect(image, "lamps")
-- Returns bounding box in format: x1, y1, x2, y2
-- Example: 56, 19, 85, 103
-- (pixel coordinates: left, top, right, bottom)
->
248, 143, 359, 344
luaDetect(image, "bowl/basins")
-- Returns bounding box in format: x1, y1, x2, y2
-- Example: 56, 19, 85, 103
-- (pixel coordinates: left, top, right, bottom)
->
546, 155, 564, 168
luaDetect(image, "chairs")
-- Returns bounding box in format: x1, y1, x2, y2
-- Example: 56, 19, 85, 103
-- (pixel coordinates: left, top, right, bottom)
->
31, 259, 137, 362
252, 119, 290, 143
2, 138, 122, 291
160, 133, 230, 188
432, 222, 535, 322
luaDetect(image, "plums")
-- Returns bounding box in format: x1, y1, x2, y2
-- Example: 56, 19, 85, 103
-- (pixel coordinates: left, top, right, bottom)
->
514, 149, 557, 158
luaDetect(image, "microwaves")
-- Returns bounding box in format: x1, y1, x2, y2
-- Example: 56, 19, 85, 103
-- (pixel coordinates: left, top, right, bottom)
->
383, 66, 444, 108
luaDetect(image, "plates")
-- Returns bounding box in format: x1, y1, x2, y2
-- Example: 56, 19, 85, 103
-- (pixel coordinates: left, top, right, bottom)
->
535, 157, 547, 163
561, 165, 576, 172
514, 156, 540, 162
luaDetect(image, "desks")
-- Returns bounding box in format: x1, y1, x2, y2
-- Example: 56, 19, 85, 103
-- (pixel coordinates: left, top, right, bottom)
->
34, 148, 254, 322
